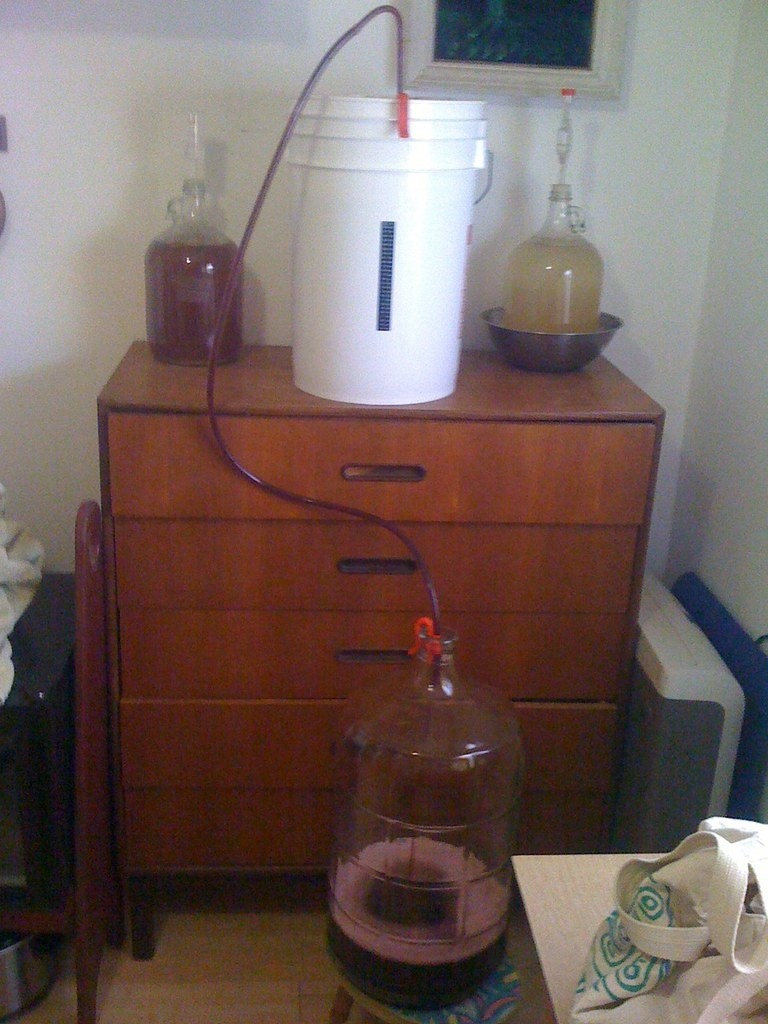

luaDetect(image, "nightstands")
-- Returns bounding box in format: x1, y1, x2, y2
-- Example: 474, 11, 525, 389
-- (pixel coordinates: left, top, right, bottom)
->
97, 338, 664, 962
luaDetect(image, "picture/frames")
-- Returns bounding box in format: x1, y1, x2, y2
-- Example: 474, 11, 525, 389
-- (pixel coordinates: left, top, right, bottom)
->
402, 0, 634, 104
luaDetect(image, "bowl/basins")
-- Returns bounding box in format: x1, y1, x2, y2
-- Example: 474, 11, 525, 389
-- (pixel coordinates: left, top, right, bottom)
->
480, 305, 624, 371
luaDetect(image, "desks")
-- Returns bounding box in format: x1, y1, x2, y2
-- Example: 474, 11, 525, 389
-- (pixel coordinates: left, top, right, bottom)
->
510, 851, 768, 1024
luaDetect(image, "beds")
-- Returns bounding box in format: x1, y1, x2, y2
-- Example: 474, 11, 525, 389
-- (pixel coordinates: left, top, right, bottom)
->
0, 498, 123, 1024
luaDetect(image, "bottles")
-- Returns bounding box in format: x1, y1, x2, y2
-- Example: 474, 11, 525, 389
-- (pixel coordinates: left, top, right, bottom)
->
142, 172, 245, 367
504, 178, 604, 337
327, 624, 528, 1012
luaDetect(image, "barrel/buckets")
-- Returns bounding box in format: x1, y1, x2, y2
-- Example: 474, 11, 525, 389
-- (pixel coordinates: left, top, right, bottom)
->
275, 90, 491, 405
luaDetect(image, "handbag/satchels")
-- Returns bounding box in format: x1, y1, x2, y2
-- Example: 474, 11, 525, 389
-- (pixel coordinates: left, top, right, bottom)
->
566, 816, 768, 1024
